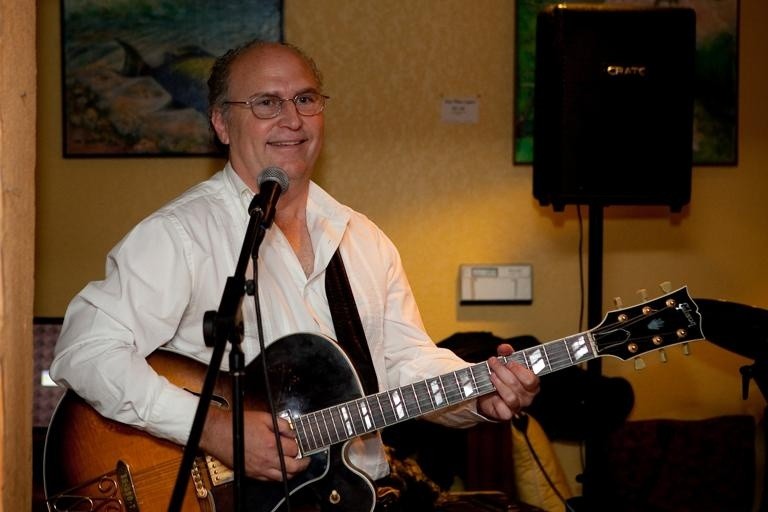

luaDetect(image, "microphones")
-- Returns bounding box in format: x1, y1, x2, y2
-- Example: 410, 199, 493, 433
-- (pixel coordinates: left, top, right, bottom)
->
250, 165, 292, 238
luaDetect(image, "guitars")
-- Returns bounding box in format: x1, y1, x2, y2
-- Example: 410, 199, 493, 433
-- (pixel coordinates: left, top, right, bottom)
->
42, 280, 710, 512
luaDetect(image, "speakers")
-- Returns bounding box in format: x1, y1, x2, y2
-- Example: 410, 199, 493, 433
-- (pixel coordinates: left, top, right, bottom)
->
531, 7, 699, 217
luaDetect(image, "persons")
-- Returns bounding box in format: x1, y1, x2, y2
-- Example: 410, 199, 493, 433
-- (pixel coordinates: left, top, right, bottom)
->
47, 38, 541, 512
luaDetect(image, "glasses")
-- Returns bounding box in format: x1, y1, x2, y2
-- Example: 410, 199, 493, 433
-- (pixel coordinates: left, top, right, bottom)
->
221, 92, 329, 119
